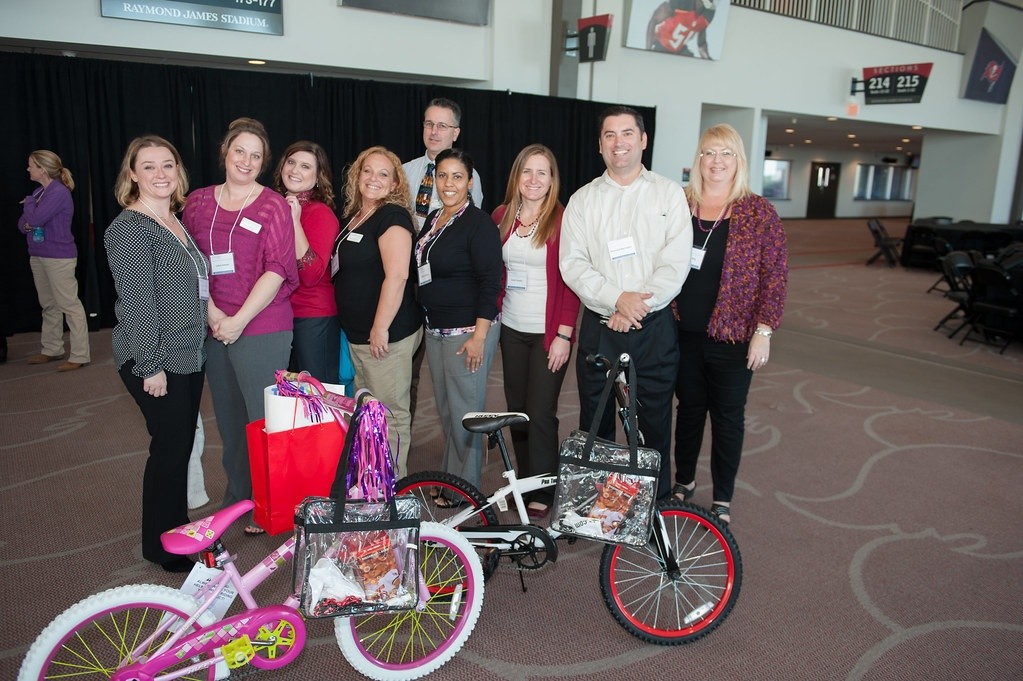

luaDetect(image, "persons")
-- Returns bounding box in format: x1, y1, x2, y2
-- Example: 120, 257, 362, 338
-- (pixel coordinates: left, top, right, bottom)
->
274, 139, 340, 383
103, 133, 210, 571
675, 124, 787, 526
645, 0, 717, 59
558, 108, 695, 499
418, 149, 501, 503
492, 143, 581, 516
20, 151, 92, 372
395, 97, 485, 450
323, 146, 425, 484
182, 117, 300, 536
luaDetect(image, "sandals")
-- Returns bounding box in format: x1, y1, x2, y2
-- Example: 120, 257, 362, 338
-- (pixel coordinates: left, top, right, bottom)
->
429, 484, 471, 508
670, 480, 697, 505
704, 502, 731, 532
244, 524, 266, 536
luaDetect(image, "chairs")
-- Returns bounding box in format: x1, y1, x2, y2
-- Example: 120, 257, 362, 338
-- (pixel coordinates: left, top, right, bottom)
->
928, 244, 1023, 356
864, 216, 903, 266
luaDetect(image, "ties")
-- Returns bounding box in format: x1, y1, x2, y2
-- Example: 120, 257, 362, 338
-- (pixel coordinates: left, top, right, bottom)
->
415, 163, 438, 219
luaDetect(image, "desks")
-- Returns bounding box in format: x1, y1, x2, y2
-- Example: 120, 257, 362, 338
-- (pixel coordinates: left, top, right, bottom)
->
900, 219, 1023, 269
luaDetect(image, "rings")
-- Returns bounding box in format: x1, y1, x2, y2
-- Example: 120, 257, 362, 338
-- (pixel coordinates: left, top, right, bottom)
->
761, 357, 765, 362
224, 342, 228, 346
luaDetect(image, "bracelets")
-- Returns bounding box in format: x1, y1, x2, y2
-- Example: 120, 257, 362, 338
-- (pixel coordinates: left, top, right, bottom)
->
754, 328, 772, 338
556, 333, 572, 342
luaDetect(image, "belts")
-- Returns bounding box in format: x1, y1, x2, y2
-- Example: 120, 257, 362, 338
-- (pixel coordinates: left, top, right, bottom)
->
585, 305, 668, 330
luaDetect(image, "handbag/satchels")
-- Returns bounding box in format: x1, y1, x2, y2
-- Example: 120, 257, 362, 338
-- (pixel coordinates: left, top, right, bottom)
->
293, 392, 431, 618
246, 388, 349, 538
547, 352, 664, 547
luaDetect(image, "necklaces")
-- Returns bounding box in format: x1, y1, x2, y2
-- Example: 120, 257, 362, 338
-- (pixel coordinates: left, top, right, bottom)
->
514, 202, 549, 238
696, 199, 730, 230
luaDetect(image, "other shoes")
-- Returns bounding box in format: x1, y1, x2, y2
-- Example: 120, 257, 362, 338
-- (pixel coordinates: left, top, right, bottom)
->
57, 357, 89, 371
506, 495, 550, 522
163, 552, 194, 572
26, 351, 64, 363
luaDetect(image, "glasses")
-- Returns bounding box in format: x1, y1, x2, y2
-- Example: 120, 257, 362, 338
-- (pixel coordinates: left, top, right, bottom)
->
699, 147, 738, 160
423, 120, 458, 131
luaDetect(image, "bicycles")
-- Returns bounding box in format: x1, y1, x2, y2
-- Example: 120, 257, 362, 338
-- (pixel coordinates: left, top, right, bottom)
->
389, 353, 746, 646
2, 370, 486, 679
